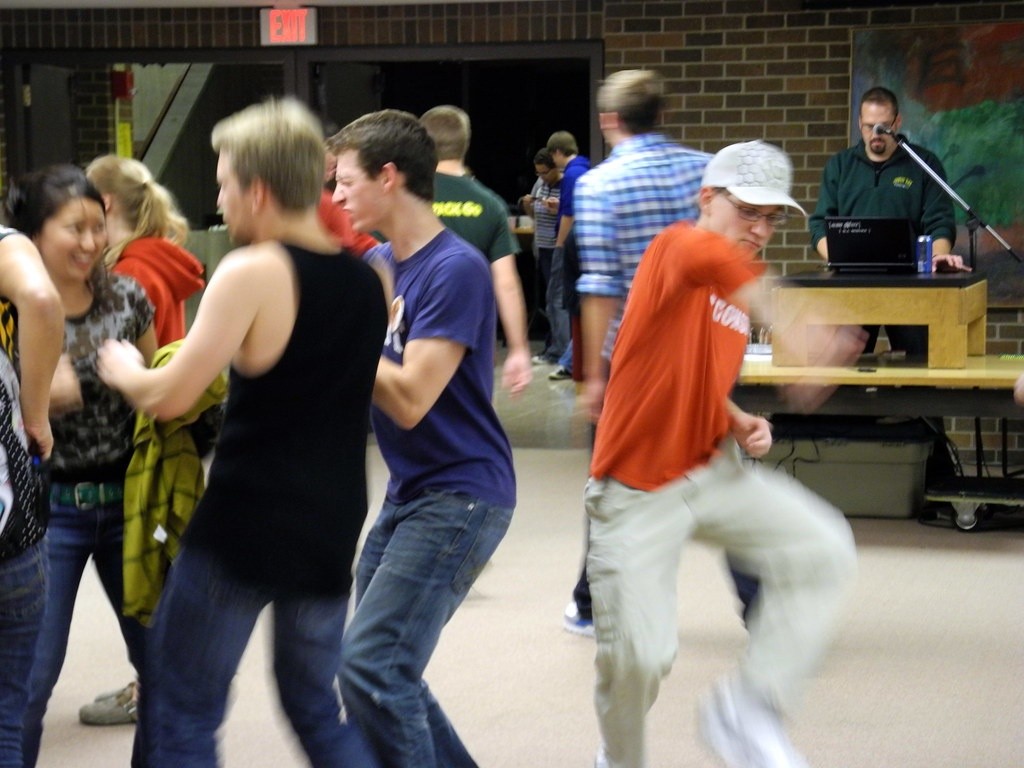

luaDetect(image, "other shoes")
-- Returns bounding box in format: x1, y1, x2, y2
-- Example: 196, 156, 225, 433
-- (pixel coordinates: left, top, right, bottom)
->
561, 605, 594, 636
550, 367, 572, 379
702, 673, 801, 768
81, 684, 141, 727
533, 353, 553, 365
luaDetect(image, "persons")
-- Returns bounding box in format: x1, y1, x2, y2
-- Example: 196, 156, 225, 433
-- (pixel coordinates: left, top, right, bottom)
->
808, 87, 963, 479
324, 111, 516, 768
319, 152, 377, 255
522, 132, 588, 378
95, 103, 390, 768
420, 106, 530, 395
561, 72, 756, 636
0, 156, 206, 766
586, 140, 853, 768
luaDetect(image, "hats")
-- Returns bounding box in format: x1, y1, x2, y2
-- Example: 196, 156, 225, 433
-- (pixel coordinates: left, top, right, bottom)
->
702, 140, 812, 216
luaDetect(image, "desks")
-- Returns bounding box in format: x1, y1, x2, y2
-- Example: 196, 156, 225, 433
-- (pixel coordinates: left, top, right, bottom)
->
737, 348, 1024, 526
774, 274, 989, 369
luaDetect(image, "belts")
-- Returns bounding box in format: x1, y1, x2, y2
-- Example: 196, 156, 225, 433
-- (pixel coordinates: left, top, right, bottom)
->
49, 483, 122, 506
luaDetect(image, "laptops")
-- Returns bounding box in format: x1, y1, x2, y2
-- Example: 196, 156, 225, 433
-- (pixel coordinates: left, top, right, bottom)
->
824, 216, 919, 274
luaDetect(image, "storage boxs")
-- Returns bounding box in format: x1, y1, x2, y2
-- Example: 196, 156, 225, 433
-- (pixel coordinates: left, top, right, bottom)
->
754, 413, 943, 519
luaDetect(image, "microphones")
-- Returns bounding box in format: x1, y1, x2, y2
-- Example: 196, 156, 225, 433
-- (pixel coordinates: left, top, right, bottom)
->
873, 124, 893, 134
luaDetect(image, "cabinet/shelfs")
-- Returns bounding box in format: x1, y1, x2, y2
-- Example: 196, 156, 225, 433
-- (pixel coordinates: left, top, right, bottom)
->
5, 63, 78, 178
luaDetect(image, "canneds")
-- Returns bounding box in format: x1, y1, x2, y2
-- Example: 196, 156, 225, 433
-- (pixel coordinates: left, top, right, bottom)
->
915, 234, 932, 273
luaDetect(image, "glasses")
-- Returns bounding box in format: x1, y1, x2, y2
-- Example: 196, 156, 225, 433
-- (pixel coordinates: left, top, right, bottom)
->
535, 171, 551, 176
863, 113, 897, 130
723, 191, 793, 227
